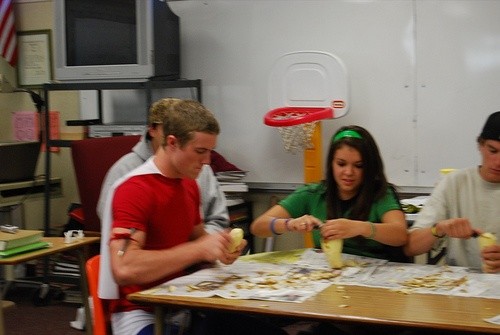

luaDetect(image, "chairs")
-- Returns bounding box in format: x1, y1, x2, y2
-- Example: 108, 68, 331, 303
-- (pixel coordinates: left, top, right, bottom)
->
86, 254, 114, 335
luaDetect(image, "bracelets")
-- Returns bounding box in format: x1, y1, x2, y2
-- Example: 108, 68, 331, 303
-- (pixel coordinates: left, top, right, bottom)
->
271, 216, 291, 236
430, 223, 446, 238
365, 221, 376, 239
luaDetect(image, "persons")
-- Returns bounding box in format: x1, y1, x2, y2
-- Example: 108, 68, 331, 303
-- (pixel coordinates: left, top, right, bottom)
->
109, 99, 249, 335
404, 114, 496, 271
95, 98, 230, 300
249, 127, 409, 263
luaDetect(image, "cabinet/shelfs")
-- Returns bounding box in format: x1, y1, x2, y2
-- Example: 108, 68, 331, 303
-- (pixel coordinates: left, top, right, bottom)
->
221, 190, 255, 259
43, 78, 202, 285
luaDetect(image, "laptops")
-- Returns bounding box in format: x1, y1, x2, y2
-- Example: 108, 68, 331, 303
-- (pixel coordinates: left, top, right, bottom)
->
0, 141, 39, 184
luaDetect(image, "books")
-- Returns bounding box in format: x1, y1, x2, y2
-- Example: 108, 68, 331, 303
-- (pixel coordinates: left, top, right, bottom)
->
0, 224, 49, 256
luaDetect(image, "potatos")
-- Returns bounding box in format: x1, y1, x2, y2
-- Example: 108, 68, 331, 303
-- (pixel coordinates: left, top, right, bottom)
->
478, 232, 497, 273
320, 234, 343, 268
227, 228, 244, 254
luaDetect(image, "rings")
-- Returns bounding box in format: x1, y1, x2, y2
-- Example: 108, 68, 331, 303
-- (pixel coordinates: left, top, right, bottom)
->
300, 223, 307, 225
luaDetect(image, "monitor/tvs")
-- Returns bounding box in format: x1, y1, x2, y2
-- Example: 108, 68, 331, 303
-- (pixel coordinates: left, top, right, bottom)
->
51, 0, 180, 83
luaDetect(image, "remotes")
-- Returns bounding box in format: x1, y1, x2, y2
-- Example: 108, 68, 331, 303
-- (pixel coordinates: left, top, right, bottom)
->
0, 224, 19, 233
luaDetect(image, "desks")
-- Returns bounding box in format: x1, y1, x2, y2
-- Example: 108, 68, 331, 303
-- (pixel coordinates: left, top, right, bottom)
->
0, 237, 102, 335
404, 212, 448, 265
127, 259, 500, 335
